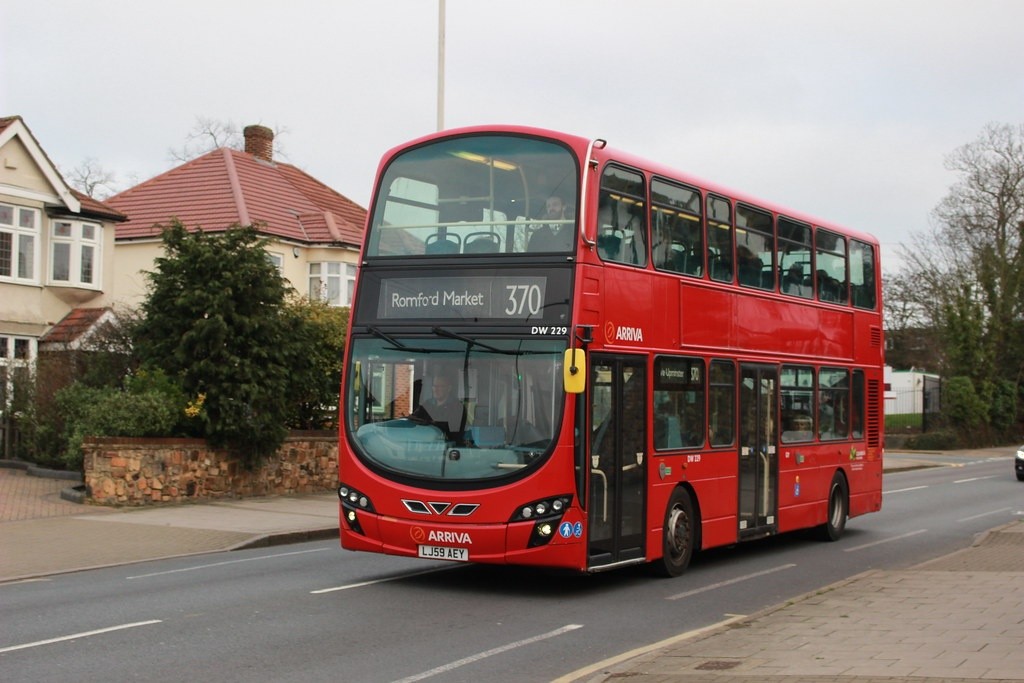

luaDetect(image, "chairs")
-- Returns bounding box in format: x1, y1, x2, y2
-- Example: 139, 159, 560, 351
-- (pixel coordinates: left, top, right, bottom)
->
416, 230, 864, 461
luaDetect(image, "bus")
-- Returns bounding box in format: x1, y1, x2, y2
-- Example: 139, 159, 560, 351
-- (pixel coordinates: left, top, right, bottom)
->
336, 124, 887, 579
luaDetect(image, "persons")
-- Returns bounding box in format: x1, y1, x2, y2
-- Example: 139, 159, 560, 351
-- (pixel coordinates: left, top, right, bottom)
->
526, 194, 576, 251
656, 362, 835, 445
411, 372, 467, 432
626, 217, 832, 303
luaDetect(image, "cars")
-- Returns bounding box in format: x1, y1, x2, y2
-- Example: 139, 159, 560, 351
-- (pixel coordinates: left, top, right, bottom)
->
1015, 444, 1024, 481
